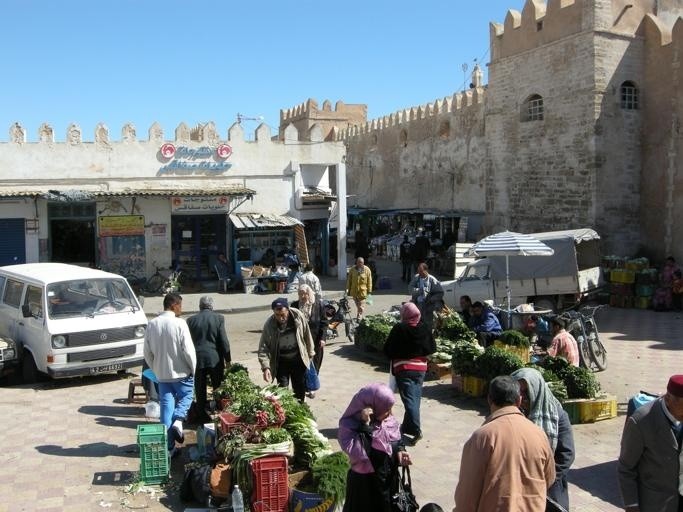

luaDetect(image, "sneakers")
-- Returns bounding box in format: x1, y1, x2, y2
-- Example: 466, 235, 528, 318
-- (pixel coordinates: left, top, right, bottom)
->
172, 420, 184, 444
168, 447, 177, 458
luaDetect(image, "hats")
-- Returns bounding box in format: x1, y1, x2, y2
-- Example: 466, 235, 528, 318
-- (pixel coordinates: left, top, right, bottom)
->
271, 298, 289, 310
667, 375, 683, 397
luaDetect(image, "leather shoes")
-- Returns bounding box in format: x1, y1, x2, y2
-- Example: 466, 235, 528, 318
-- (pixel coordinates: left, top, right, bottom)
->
410, 431, 423, 445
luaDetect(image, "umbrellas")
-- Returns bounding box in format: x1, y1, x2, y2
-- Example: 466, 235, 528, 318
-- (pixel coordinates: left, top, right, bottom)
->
464, 230, 554, 331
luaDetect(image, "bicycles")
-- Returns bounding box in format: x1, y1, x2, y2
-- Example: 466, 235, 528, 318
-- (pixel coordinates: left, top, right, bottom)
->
146, 262, 181, 293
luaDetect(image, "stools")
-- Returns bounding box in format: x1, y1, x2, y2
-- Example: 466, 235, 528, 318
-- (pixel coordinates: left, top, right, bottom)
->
128, 379, 150, 403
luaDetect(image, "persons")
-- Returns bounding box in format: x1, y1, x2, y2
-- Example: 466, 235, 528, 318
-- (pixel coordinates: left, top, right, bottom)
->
417, 503, 443, 512
329, 227, 339, 266
218, 254, 239, 292
187, 296, 232, 416
672, 268, 683, 312
413, 236, 427, 273
469, 301, 505, 347
299, 264, 322, 301
458, 295, 472, 324
143, 292, 196, 458
338, 382, 413, 512
546, 319, 579, 368
384, 303, 437, 445
652, 256, 681, 310
400, 235, 414, 281
420, 230, 431, 256
617, 375, 683, 512
258, 298, 315, 403
509, 368, 575, 512
353, 231, 368, 265
452, 377, 556, 512
291, 284, 329, 399
345, 258, 373, 321
408, 263, 445, 335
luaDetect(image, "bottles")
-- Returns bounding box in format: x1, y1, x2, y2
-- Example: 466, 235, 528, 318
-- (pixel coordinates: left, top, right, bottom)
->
232, 485, 244, 512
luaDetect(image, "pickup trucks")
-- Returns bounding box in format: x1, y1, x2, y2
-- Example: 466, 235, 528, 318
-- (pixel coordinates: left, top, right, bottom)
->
441, 260, 604, 310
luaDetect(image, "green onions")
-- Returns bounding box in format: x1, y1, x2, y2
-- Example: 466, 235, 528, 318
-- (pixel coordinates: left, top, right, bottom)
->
229, 440, 294, 494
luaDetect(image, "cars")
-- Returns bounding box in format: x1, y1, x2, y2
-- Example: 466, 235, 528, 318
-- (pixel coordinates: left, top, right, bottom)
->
0, 335, 18, 370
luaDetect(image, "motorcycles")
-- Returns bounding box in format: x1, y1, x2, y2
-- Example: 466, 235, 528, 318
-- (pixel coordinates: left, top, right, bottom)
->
321, 289, 360, 342
559, 305, 608, 372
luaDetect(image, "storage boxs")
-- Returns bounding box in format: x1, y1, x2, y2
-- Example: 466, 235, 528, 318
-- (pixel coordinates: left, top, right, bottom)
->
636, 272, 657, 283
451, 374, 464, 392
429, 364, 451, 381
218, 413, 281, 434
136, 424, 171, 485
564, 403, 580, 425
624, 262, 648, 272
608, 284, 635, 296
610, 296, 633, 307
250, 455, 289, 512
600, 259, 625, 268
580, 396, 618, 424
203, 423, 217, 456
289, 470, 335, 512
635, 283, 659, 296
463, 376, 486, 401
633, 296, 653, 309
610, 268, 636, 283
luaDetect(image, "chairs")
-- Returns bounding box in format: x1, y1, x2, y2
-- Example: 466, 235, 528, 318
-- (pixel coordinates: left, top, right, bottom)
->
215, 263, 235, 294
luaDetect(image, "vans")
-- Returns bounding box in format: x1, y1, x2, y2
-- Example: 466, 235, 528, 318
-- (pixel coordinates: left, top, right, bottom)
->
0, 264, 148, 383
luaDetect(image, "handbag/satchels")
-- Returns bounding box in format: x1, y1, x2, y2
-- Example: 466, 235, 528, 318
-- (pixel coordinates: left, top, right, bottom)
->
366, 294, 373, 306
305, 359, 320, 392
